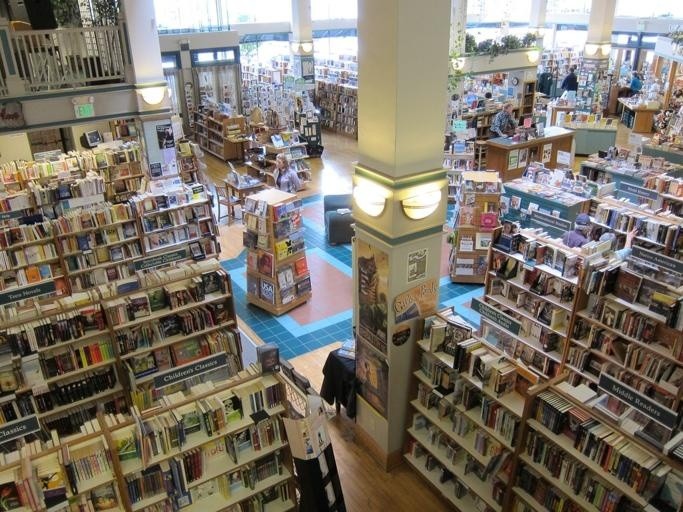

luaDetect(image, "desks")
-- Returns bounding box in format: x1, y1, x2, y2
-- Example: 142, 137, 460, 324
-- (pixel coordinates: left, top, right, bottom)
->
221, 176, 263, 222
330, 342, 356, 423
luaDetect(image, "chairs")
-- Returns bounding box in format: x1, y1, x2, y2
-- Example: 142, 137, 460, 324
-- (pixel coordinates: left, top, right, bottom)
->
212, 182, 246, 225
10, 21, 67, 91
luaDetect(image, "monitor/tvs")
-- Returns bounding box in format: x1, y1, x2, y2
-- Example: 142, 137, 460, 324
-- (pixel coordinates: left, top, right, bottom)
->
149, 161, 163, 179
518, 114, 533, 126
80, 129, 103, 149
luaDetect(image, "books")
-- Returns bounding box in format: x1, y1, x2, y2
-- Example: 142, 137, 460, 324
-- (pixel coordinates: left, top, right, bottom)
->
481, 227, 681, 414
592, 174, 682, 286
188, 49, 357, 141
1, 260, 242, 452
243, 196, 313, 305
446, 141, 475, 196
451, 181, 499, 275
0, 363, 292, 512
500, 143, 592, 223
0, 142, 221, 308
412, 310, 681, 512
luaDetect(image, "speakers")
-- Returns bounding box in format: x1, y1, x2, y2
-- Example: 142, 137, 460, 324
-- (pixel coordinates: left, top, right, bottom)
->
24, 0, 57, 30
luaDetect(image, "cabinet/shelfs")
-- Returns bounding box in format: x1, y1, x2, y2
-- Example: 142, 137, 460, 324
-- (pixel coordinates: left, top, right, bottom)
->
401, 42, 683, 512
0, 137, 222, 308
1, 258, 346, 510
272, 54, 357, 140
240, 187, 311, 318
184, 54, 324, 192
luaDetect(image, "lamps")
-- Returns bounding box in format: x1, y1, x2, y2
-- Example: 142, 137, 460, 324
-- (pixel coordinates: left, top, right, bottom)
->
289, 40, 300, 54
301, 39, 314, 53
351, 187, 386, 218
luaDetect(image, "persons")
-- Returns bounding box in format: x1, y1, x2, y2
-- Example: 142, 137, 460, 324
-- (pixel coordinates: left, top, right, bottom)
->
489, 104, 518, 141
538, 67, 553, 97
483, 93, 493, 107
275, 153, 302, 196
629, 71, 642, 97
562, 66, 577, 101
564, 214, 591, 250
